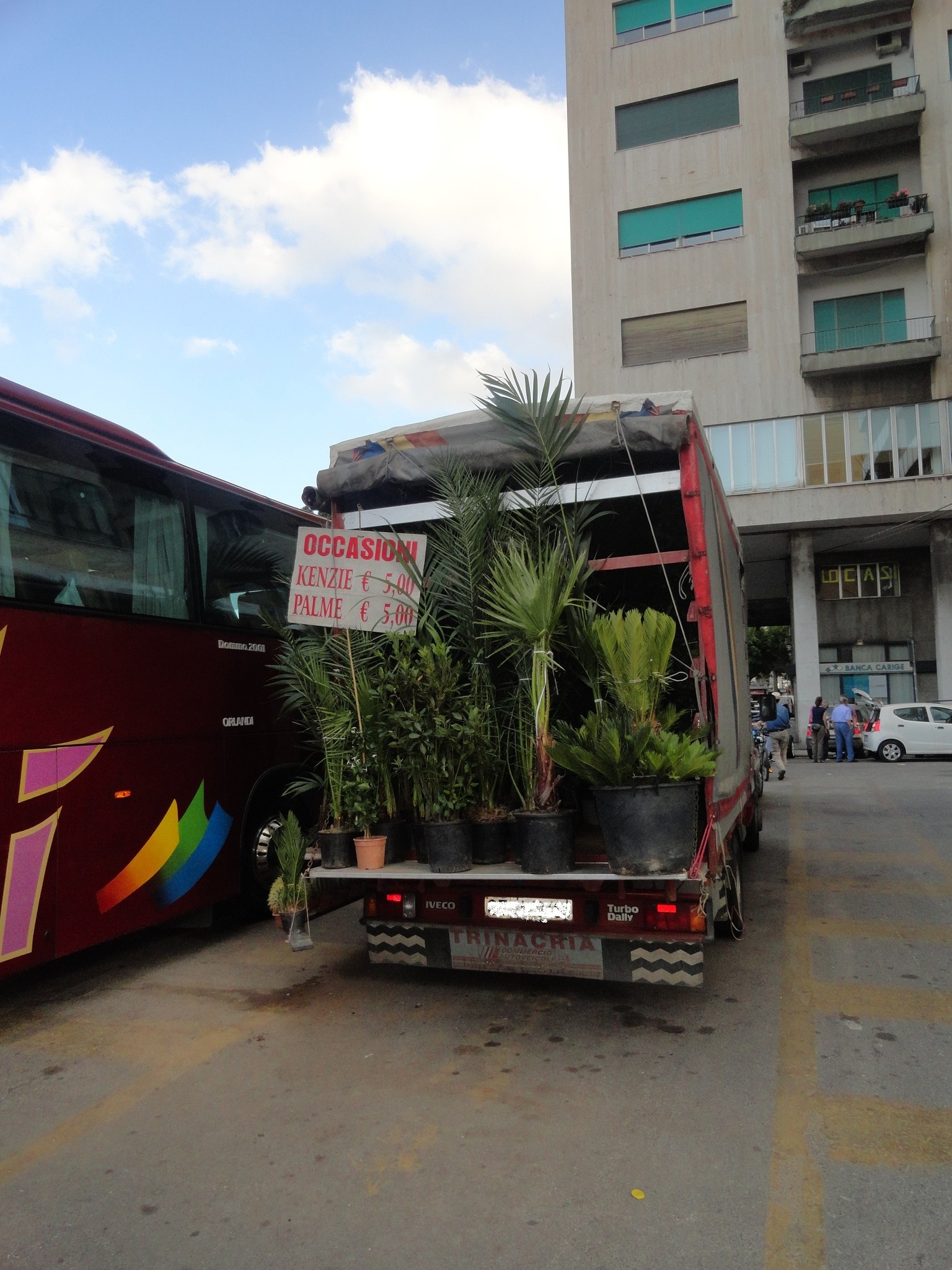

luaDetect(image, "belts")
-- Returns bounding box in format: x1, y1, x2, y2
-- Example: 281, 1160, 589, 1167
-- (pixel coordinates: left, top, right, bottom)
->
770, 729, 784, 732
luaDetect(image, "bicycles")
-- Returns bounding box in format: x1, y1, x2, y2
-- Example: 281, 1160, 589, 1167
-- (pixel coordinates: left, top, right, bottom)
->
749, 729, 771, 781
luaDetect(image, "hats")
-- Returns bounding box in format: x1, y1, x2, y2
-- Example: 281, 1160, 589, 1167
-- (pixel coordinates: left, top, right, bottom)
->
772, 692, 780, 699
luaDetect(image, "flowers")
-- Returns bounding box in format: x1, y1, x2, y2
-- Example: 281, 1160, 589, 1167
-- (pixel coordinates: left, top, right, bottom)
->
805, 199, 853, 217
885, 188, 909, 200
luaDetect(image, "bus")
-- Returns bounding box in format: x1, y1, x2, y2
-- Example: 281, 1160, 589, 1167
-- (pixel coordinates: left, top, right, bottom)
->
0, 374, 333, 988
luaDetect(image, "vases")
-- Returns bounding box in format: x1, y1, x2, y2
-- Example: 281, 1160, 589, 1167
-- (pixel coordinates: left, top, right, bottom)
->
888, 196, 909, 208
805, 207, 851, 224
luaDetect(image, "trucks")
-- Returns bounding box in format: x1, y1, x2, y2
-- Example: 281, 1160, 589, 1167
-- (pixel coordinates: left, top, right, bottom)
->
269, 389, 765, 988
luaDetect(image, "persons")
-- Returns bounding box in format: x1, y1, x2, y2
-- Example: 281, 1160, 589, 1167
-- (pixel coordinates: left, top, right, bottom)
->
761, 692, 860, 780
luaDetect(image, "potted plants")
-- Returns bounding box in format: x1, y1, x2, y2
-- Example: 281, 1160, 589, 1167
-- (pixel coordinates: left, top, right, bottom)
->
257, 370, 725, 933
854, 200, 865, 212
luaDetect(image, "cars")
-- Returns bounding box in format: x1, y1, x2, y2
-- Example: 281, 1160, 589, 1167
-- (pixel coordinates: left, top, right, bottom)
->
851, 688, 952, 763
778, 697, 795, 719
750, 700, 760, 716
806, 703, 869, 759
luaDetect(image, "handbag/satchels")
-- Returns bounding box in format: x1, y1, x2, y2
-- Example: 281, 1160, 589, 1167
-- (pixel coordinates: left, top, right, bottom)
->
812, 725, 820, 732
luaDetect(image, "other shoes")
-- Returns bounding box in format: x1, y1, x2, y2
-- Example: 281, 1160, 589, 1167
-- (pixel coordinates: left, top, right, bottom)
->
849, 759, 859, 762
778, 769, 786, 780
812, 760, 826, 763
837, 760, 843, 762
